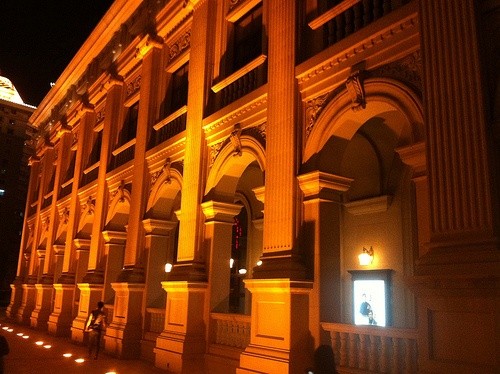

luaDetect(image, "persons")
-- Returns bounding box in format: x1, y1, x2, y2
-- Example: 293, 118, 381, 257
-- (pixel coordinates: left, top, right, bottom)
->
366, 310, 377, 326
359, 293, 371, 315
308, 343, 340, 374
83, 301, 109, 360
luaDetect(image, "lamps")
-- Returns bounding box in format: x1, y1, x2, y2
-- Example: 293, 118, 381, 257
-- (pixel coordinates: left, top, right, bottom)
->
358, 247, 374, 266
165, 262, 172, 273
230, 258, 262, 275
